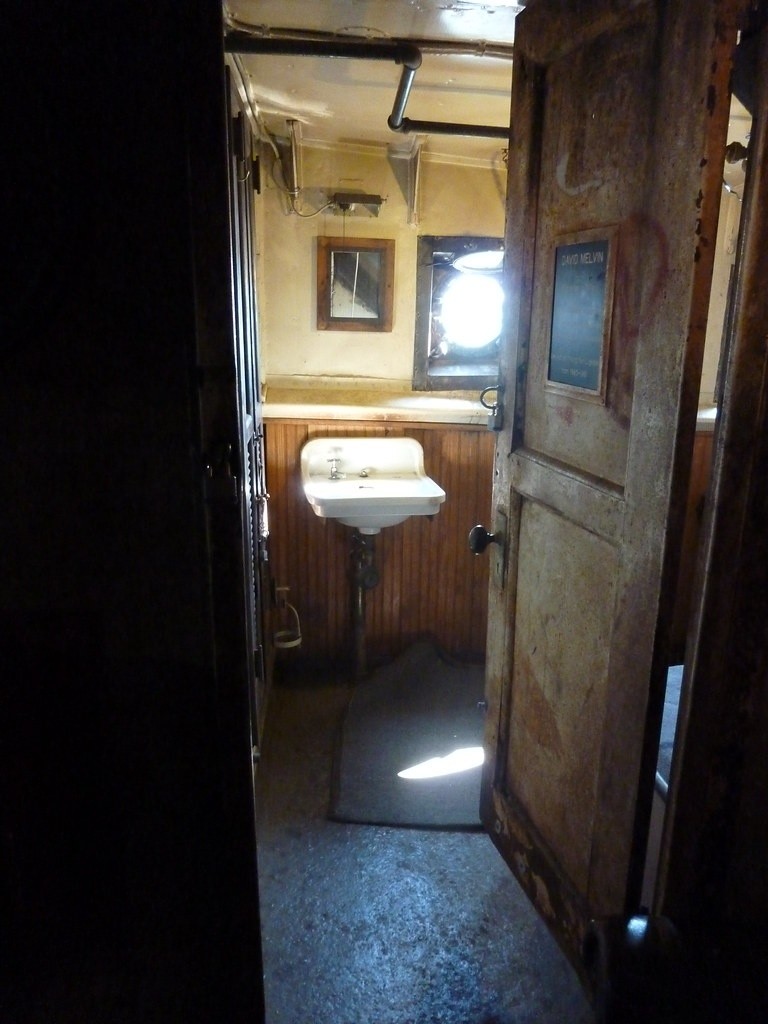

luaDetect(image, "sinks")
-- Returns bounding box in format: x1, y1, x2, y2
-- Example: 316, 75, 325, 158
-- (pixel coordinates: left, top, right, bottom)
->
304, 480, 446, 529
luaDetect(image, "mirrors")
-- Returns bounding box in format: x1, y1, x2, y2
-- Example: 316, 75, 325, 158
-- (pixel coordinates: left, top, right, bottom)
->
317, 234, 396, 331
412, 236, 505, 393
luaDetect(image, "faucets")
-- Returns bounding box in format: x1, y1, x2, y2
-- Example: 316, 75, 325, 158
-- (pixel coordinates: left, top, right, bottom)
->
327, 458, 345, 478
